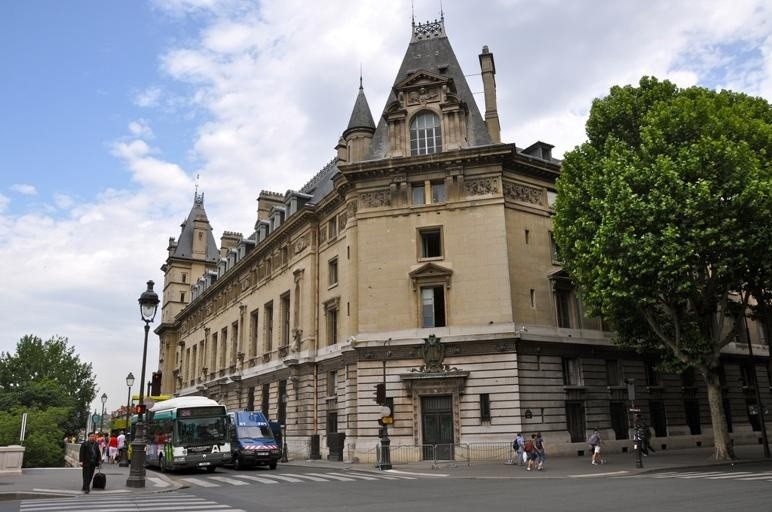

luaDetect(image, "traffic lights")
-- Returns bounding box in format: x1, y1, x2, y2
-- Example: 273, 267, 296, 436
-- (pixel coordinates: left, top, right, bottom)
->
373, 384, 385, 405
623, 377, 636, 401
136, 404, 147, 415
151, 371, 162, 396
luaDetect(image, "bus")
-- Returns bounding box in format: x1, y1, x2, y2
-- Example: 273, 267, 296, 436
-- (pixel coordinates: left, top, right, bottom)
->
130, 395, 233, 473
109, 414, 137, 463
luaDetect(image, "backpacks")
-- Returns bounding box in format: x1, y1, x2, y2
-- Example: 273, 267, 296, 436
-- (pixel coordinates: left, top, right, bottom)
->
513, 439, 520, 452
525, 440, 534, 453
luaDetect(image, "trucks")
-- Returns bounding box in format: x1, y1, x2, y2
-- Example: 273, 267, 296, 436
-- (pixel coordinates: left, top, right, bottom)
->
217, 407, 283, 472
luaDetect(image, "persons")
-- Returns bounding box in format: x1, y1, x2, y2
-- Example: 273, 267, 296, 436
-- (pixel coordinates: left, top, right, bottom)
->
535, 434, 545, 469
641, 422, 656, 456
64, 430, 126, 464
515, 433, 527, 465
526, 434, 542, 470
589, 427, 601, 465
80, 432, 102, 493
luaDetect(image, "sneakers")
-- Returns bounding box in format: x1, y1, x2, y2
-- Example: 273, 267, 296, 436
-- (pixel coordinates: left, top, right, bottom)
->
527, 466, 544, 471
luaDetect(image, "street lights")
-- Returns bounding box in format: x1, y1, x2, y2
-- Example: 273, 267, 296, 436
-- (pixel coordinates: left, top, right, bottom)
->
92, 409, 98, 434
119, 371, 135, 467
374, 337, 394, 470
126, 278, 160, 489
101, 392, 108, 432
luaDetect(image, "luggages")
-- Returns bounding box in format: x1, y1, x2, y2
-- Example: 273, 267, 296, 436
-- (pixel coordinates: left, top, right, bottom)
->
92, 466, 106, 489
